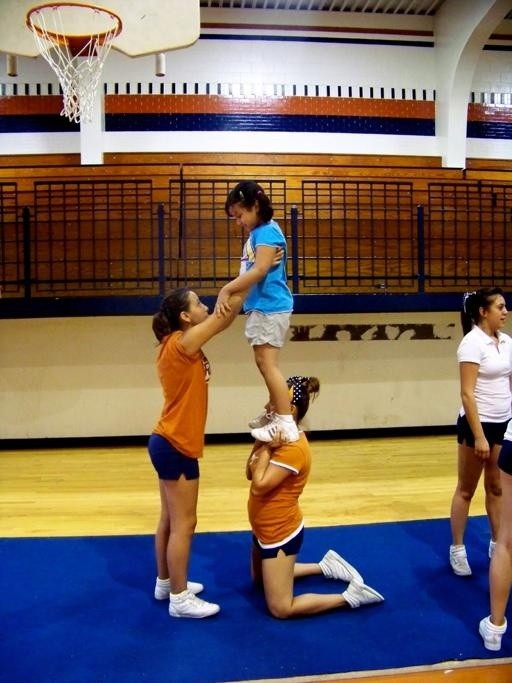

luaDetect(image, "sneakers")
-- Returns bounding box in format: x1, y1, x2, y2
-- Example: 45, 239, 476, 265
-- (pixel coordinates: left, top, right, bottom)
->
169, 589, 221, 618
449, 544, 472, 576
478, 614, 507, 652
154, 576, 204, 600
342, 577, 385, 608
488, 538, 497, 558
319, 549, 365, 583
248, 409, 300, 444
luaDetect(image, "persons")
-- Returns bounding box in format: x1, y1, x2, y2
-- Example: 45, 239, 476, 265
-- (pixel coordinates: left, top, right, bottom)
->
447, 286, 511, 577
145, 244, 287, 620
478, 421, 511, 652
244, 375, 387, 620
211, 180, 301, 444
